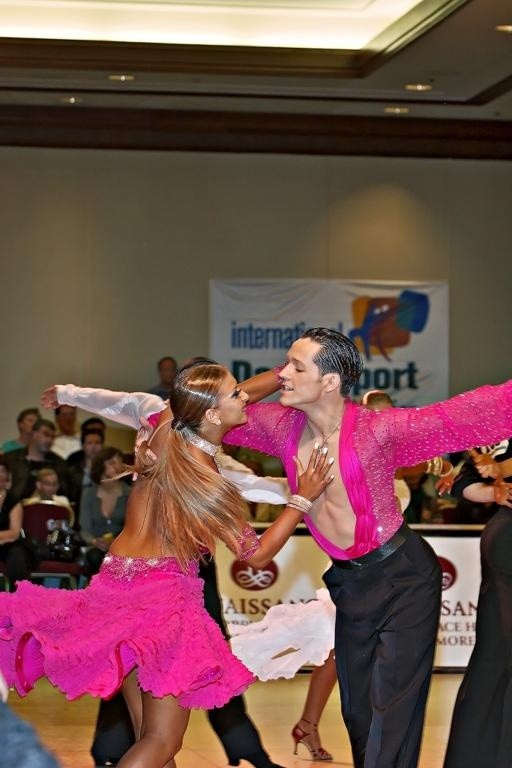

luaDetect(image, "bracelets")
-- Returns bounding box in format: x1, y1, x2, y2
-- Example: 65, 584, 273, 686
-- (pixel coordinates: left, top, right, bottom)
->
288, 494, 313, 513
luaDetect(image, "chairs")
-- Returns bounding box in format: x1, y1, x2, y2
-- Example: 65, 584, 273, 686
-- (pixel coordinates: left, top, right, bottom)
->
20, 501, 81, 590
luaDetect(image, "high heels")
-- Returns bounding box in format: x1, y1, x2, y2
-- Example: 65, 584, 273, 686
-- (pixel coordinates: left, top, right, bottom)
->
228, 751, 286, 768
93, 753, 117, 767
293, 719, 333, 761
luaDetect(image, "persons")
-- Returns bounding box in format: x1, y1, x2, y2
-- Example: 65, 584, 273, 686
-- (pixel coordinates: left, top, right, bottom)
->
443, 498, 511, 768
40, 355, 278, 767
146, 357, 178, 401
294, 390, 454, 760
1, 404, 132, 591
200, 449, 457, 762
403, 435, 508, 525
133, 324, 511, 767
108, 363, 338, 766
240, 455, 283, 523
